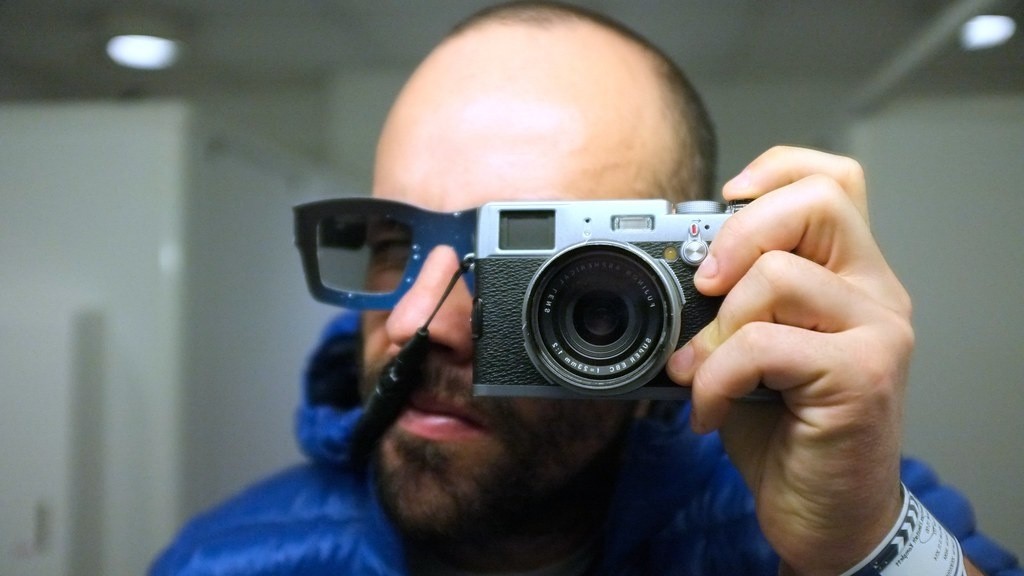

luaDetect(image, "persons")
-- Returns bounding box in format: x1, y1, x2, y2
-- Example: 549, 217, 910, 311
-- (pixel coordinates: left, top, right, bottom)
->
140, 0, 1024, 576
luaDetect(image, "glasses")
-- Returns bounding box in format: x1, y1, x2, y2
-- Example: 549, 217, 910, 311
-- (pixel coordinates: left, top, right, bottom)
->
295, 197, 480, 311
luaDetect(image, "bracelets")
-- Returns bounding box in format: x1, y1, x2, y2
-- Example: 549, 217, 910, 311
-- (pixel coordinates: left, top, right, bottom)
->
829, 479, 966, 576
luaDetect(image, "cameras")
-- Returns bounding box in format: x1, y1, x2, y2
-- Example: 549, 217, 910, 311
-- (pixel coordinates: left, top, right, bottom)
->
470, 199, 768, 402
